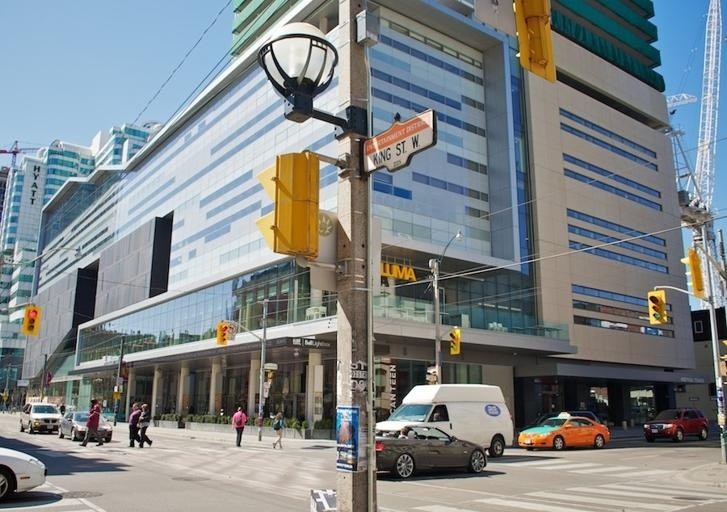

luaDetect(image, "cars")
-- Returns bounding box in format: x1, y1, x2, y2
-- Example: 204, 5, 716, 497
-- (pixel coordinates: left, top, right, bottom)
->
374, 419, 488, 481
516, 414, 613, 452
0, 445, 49, 502
57, 410, 113, 443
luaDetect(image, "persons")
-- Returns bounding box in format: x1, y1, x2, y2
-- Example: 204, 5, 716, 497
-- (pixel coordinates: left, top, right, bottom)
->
127, 402, 141, 447
230, 406, 248, 448
58, 402, 66, 415
137, 403, 153, 448
270, 411, 284, 449
77, 397, 104, 447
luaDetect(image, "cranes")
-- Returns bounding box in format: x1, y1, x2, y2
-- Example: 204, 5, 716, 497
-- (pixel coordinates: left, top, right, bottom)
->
666, 91, 698, 111
670, 0, 727, 312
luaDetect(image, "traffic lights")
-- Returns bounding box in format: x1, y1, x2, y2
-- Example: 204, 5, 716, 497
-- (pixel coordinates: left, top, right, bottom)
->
679, 248, 706, 299
21, 306, 41, 336
647, 290, 668, 325
450, 329, 460, 355
216, 321, 229, 346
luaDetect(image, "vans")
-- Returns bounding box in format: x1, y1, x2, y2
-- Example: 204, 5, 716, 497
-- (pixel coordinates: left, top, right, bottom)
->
387, 380, 518, 459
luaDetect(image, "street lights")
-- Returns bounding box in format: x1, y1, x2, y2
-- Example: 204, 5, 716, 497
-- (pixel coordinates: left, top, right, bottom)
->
429, 230, 465, 383
260, 20, 377, 512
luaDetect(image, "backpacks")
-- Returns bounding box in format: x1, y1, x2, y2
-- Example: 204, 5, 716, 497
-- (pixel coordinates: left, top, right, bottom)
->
273, 420, 281, 430
236, 412, 243, 426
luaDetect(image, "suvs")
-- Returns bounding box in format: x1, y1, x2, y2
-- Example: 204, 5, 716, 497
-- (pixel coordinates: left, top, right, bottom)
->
19, 400, 63, 435
642, 406, 712, 443
